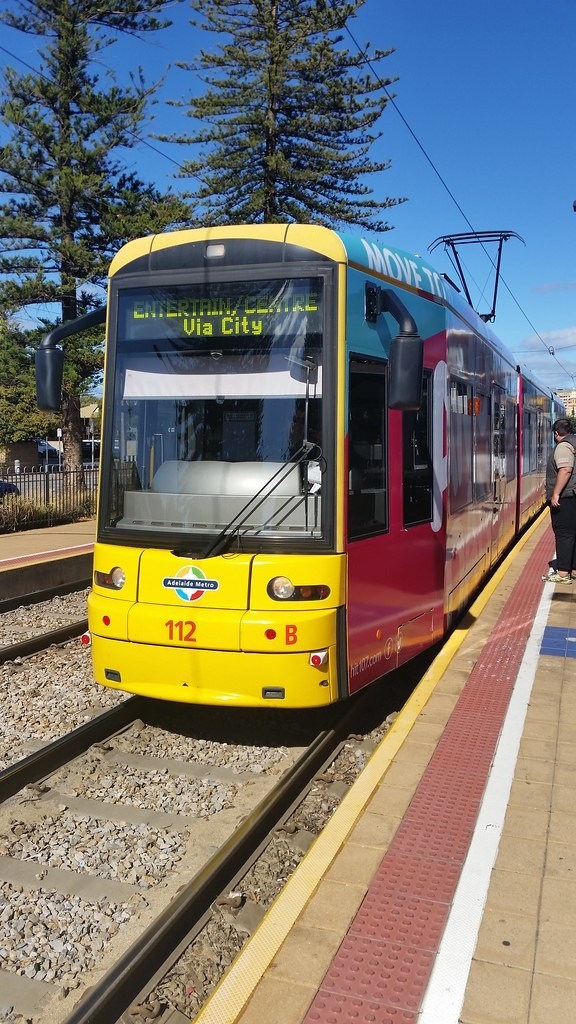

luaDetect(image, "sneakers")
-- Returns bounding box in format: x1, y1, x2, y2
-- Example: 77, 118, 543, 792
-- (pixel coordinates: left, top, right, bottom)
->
553, 569, 576, 578
542, 570, 572, 585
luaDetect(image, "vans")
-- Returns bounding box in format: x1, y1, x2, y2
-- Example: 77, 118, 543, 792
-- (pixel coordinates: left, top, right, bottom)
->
81, 439, 101, 462
38, 440, 57, 458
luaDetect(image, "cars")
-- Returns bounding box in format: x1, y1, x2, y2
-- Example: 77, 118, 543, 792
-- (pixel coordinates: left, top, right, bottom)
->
0, 480, 19, 503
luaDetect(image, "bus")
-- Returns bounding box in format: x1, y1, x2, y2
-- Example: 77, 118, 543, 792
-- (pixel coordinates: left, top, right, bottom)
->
34, 230, 567, 712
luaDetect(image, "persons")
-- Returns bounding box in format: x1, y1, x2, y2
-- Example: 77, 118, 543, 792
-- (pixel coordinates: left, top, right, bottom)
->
542, 418, 576, 584
